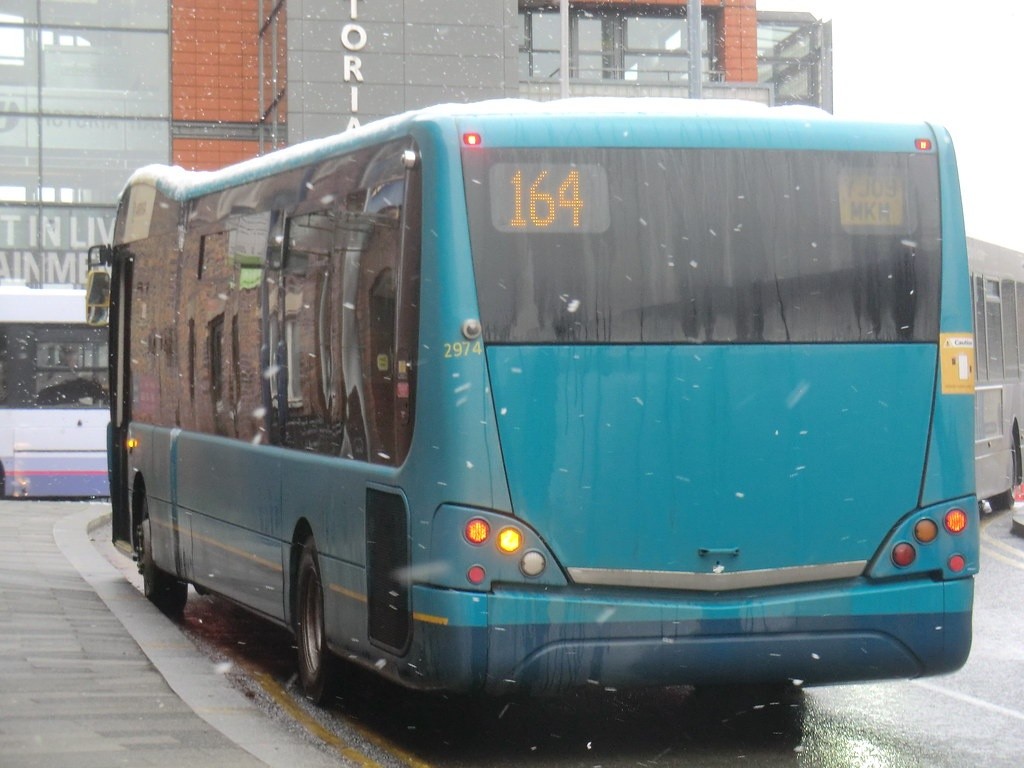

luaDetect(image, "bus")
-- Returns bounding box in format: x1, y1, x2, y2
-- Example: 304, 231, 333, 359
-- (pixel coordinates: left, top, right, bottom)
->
963, 234, 1024, 526
0, 282, 120, 503
84, 96, 981, 724
